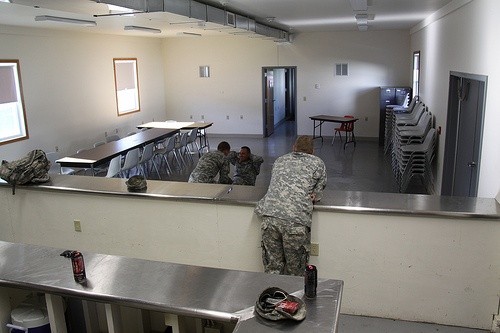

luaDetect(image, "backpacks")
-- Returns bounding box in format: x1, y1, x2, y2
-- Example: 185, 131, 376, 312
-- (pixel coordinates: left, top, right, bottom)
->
0, 149, 51, 187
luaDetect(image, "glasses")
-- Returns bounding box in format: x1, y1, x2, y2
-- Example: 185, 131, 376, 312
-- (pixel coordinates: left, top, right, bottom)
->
239, 153, 244, 154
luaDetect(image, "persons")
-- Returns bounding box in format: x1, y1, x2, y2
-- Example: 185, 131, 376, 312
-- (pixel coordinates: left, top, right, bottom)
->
228, 146, 264, 186
255, 136, 328, 276
187, 141, 230, 184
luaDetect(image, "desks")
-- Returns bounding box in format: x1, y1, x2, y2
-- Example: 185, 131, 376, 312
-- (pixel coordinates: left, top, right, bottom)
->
137, 121, 213, 157
0, 239, 344, 333
308, 115, 359, 151
53, 127, 179, 174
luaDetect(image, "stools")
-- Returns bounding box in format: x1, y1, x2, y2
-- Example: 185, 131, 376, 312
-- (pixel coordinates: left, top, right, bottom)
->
9, 304, 50, 333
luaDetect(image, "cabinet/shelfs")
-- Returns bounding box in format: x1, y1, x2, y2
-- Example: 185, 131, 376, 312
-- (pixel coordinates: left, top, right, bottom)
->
378, 86, 411, 147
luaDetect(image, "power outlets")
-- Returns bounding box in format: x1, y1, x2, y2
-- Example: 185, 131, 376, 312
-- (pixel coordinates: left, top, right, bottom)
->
74, 220, 82, 232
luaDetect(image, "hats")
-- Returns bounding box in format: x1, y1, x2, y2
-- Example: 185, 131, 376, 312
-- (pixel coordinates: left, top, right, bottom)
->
255, 286, 307, 321
126, 174, 147, 190
295, 135, 314, 153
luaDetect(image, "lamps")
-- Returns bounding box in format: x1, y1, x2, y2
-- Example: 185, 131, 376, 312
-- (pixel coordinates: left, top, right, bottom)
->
34, 16, 97, 28
359, 25, 368, 31
178, 33, 201, 36
124, 26, 161, 33
351, 0, 367, 11
355, 14, 367, 26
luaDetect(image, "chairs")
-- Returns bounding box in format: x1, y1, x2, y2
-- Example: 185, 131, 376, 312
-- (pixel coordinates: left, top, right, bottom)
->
331, 116, 354, 144
385, 93, 439, 193
41, 127, 199, 180
